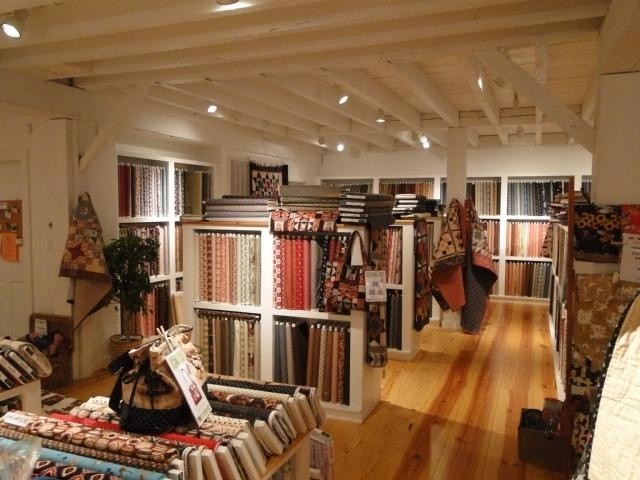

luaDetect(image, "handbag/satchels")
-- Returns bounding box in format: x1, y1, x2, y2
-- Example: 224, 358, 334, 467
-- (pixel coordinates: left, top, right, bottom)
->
108, 325, 210, 434
330, 264, 374, 310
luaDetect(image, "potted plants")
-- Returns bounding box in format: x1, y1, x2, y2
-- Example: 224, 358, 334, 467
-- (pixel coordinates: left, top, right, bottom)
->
105, 229, 162, 363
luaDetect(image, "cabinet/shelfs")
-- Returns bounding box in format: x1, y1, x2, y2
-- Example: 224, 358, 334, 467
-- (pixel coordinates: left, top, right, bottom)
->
180, 143, 502, 422
505, 175, 572, 305
263, 424, 320, 478
115, 142, 218, 341
2, 377, 45, 415
